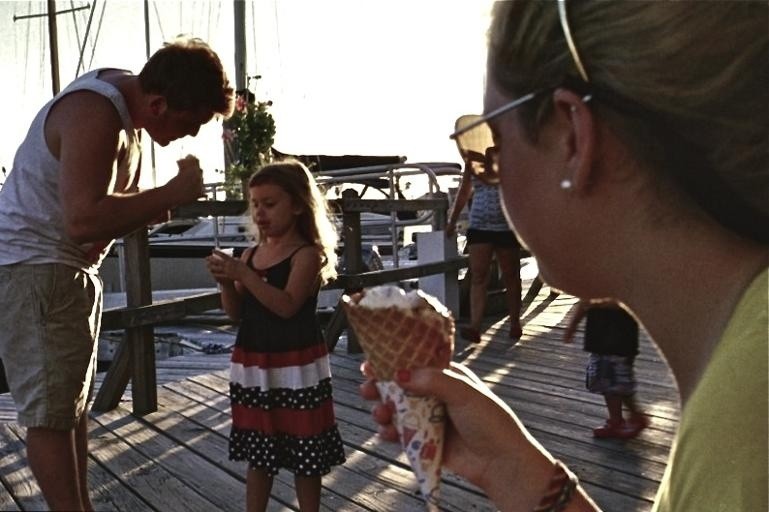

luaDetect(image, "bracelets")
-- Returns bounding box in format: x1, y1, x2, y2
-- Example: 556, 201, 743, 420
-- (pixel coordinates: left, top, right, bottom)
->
535, 458, 578, 512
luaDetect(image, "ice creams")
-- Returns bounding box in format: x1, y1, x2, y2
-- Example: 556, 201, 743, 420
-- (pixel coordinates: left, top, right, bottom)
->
176, 154, 199, 168
341, 286, 455, 382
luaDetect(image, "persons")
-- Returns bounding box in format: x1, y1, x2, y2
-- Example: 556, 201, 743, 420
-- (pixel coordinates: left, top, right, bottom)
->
348, 1, 768, 512
0, 29, 236, 510
442, 154, 528, 343
203, 155, 346, 511
560, 298, 653, 442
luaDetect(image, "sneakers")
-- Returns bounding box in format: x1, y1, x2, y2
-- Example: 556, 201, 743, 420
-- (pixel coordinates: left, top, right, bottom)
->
509, 323, 522, 338
593, 417, 625, 439
457, 324, 481, 343
616, 413, 648, 438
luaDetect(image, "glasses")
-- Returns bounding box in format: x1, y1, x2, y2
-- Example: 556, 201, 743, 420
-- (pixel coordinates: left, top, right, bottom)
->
449, 82, 589, 189
